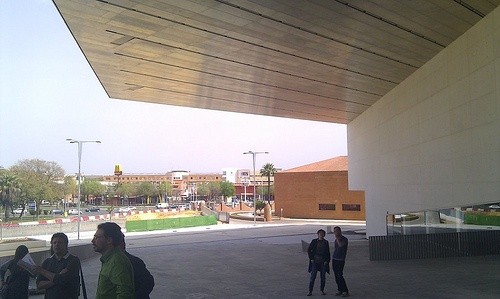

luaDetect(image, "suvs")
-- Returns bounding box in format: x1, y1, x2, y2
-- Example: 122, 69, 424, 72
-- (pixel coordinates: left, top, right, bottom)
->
68, 209, 82, 215
11, 208, 27, 214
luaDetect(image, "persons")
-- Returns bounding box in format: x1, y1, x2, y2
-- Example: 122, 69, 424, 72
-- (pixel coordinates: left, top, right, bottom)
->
122, 237, 155, 299
332, 226, 350, 297
0, 245, 35, 299
91, 222, 135, 299
33, 233, 80, 299
307, 229, 331, 295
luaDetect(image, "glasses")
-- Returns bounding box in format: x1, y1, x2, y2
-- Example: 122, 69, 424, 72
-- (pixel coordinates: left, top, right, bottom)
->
93, 235, 106, 241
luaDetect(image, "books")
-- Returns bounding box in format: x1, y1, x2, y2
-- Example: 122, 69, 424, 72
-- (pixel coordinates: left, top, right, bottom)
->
17, 253, 37, 277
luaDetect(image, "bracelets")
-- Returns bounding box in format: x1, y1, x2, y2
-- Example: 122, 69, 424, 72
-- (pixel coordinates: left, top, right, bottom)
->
0, 280, 4, 281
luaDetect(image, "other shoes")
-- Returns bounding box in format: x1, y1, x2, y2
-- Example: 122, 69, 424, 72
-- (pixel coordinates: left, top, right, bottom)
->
342, 292, 349, 296
320, 289, 326, 295
336, 292, 342, 296
306, 291, 312, 296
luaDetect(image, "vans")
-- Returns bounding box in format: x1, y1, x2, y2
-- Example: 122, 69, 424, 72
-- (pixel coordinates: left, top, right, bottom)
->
52, 210, 64, 215
156, 203, 169, 209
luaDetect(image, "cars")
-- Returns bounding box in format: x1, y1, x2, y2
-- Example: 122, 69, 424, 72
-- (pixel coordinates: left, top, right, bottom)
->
86, 207, 100, 212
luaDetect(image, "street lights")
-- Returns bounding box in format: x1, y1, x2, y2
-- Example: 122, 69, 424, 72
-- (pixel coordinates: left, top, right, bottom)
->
243, 151, 269, 224
66, 138, 102, 240
